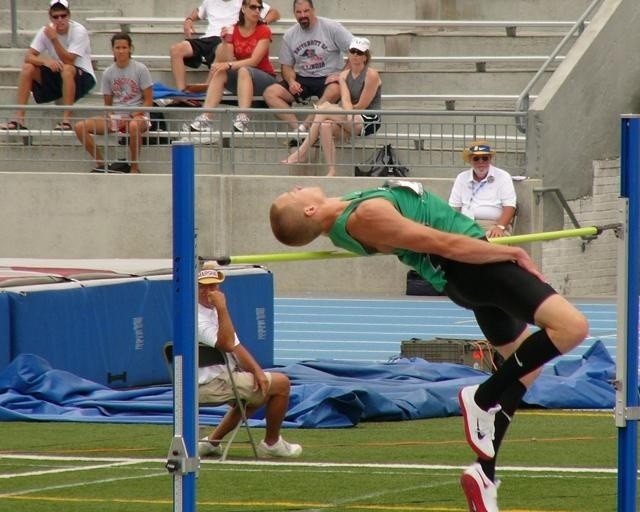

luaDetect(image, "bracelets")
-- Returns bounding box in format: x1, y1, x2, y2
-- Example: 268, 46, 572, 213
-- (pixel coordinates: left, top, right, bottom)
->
185, 17, 193, 22
495, 224, 507, 232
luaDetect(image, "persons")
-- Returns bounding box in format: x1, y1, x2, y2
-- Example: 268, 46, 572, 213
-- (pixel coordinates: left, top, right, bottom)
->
282, 37, 383, 176
169, 0, 280, 108
270, 182, 588, 512
194, 263, 301, 459
0, 0, 96, 130
446, 141, 517, 238
263, 0, 355, 147
75, 34, 154, 175
188, 1, 277, 133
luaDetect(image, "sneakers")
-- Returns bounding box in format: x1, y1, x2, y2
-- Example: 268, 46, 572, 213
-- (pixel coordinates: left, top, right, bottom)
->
256, 434, 304, 460
454, 383, 504, 462
197, 435, 225, 457
457, 461, 502, 512
232, 113, 251, 132
189, 114, 215, 134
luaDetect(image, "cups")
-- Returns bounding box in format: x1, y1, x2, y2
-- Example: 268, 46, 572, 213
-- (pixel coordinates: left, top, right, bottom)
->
109, 116, 121, 131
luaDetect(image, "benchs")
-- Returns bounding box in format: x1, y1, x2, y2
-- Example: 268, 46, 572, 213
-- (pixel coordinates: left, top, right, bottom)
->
1, 12, 591, 156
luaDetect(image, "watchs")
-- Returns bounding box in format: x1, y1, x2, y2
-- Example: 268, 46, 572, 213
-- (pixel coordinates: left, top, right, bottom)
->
227, 61, 232, 72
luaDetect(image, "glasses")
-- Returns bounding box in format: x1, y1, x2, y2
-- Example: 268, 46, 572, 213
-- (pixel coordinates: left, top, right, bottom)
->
244, 5, 264, 12
470, 156, 490, 162
49, 14, 69, 19
349, 49, 366, 56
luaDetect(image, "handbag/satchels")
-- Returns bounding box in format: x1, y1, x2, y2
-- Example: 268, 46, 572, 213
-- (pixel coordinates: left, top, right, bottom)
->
354, 143, 409, 178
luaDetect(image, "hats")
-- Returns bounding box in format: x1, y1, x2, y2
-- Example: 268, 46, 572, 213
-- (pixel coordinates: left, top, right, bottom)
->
47, 0, 71, 10
196, 264, 228, 286
463, 140, 497, 164
349, 37, 371, 52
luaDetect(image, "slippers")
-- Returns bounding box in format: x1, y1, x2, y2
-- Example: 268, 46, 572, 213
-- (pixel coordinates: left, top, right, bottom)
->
54, 122, 72, 131
0, 120, 29, 130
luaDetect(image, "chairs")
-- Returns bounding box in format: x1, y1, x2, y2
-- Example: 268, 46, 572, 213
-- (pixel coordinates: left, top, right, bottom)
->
507, 204, 524, 236
159, 339, 262, 463
